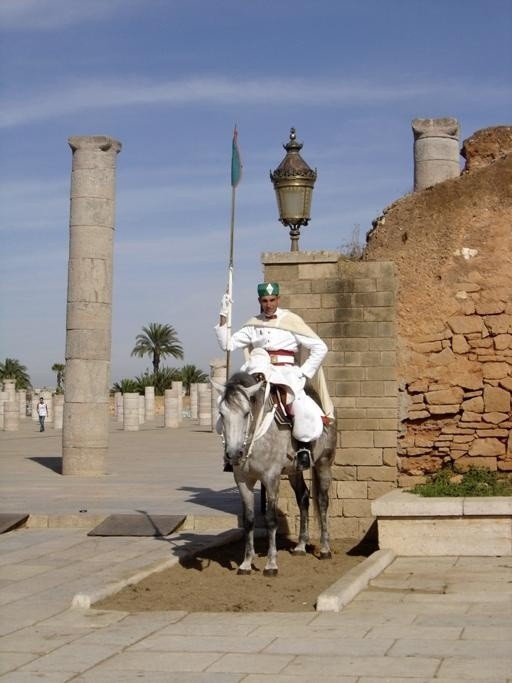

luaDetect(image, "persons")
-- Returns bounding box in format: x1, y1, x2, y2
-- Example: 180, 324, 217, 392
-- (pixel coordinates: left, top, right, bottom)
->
214, 282, 329, 472
36, 397, 48, 432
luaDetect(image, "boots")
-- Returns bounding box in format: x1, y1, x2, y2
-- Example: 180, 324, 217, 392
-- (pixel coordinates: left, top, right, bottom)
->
295, 442, 311, 470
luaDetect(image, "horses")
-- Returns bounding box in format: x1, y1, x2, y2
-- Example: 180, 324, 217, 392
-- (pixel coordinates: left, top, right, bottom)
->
207, 374, 341, 577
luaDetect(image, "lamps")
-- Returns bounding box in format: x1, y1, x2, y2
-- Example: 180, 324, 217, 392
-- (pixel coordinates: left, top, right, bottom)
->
267, 121, 320, 253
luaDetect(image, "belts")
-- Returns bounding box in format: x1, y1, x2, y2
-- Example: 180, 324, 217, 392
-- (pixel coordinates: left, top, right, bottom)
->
268, 355, 294, 364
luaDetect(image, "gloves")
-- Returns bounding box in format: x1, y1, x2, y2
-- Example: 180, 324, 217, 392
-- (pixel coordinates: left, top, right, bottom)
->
219, 293, 233, 317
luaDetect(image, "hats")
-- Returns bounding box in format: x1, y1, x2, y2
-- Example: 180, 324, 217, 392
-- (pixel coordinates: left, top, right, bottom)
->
258, 283, 279, 295
